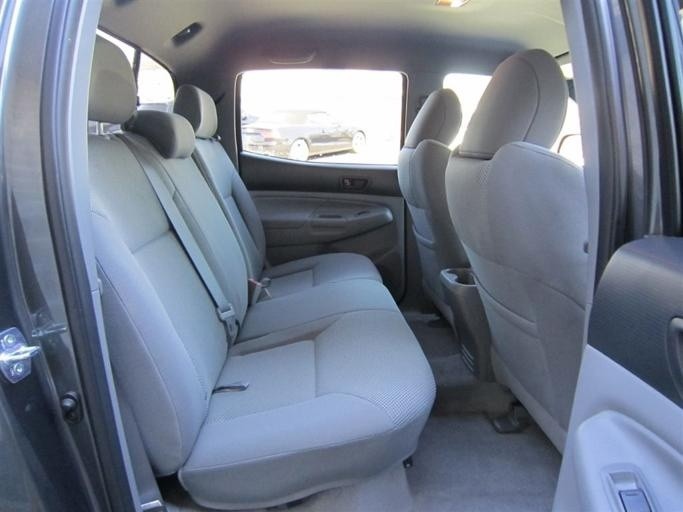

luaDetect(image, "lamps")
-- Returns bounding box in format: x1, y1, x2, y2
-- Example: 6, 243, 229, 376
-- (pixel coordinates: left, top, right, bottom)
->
434, 0, 471, 11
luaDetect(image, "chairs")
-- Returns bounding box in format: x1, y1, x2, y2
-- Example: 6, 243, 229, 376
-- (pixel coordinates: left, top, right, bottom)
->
444, 48, 597, 454
81, 32, 438, 512
116, 111, 401, 345
173, 83, 382, 303
392, 89, 473, 334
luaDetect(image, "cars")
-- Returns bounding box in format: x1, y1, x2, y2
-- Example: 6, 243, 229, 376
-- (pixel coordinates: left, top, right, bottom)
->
241, 108, 372, 162
136, 99, 173, 114
